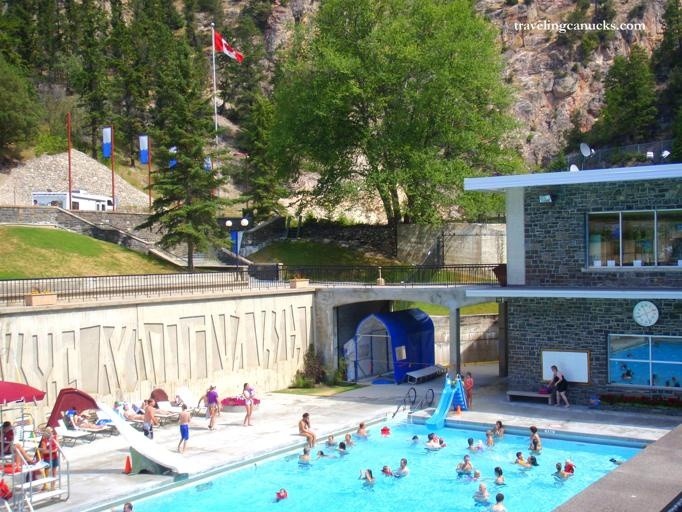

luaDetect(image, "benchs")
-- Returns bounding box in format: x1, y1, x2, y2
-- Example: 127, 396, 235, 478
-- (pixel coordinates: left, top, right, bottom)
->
405, 366, 447, 385
289, 278, 309, 289
506, 390, 556, 405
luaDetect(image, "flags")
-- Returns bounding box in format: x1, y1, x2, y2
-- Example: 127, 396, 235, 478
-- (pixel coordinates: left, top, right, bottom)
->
214, 30, 244, 63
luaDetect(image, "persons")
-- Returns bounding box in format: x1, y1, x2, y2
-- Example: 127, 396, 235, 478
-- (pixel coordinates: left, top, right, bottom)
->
410, 433, 448, 451
457, 421, 542, 512
177, 404, 191, 454
552, 459, 576, 481
609, 458, 624, 465
73, 410, 105, 429
547, 365, 570, 408
381, 458, 409, 480
0, 421, 17, 454
456, 371, 474, 410
123, 502, 134, 512
123, 397, 160, 440
359, 469, 375, 485
619, 352, 681, 388
274, 489, 287, 502
197, 385, 222, 431
381, 426, 392, 437
42, 426, 59, 490
242, 383, 254, 427
297, 411, 368, 463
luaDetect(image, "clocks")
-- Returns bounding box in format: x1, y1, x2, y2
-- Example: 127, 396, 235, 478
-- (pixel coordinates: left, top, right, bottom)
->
632, 301, 660, 327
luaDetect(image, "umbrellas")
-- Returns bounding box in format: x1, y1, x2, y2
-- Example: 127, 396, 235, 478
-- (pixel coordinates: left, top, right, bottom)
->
0, 380, 46, 464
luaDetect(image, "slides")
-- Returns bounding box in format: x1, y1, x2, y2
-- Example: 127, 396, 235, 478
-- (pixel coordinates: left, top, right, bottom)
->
424, 375, 467, 429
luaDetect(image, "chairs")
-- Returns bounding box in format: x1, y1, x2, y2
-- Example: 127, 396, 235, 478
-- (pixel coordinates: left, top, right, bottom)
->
12, 400, 202, 454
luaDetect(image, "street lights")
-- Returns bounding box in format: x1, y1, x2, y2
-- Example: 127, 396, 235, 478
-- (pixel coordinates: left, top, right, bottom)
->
225, 218, 251, 281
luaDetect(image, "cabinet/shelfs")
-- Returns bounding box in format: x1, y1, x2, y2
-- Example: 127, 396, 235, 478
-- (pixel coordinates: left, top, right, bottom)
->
589, 233, 612, 265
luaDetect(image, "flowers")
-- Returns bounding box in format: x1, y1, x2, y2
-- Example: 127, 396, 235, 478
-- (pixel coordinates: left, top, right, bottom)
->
221, 397, 261, 405
